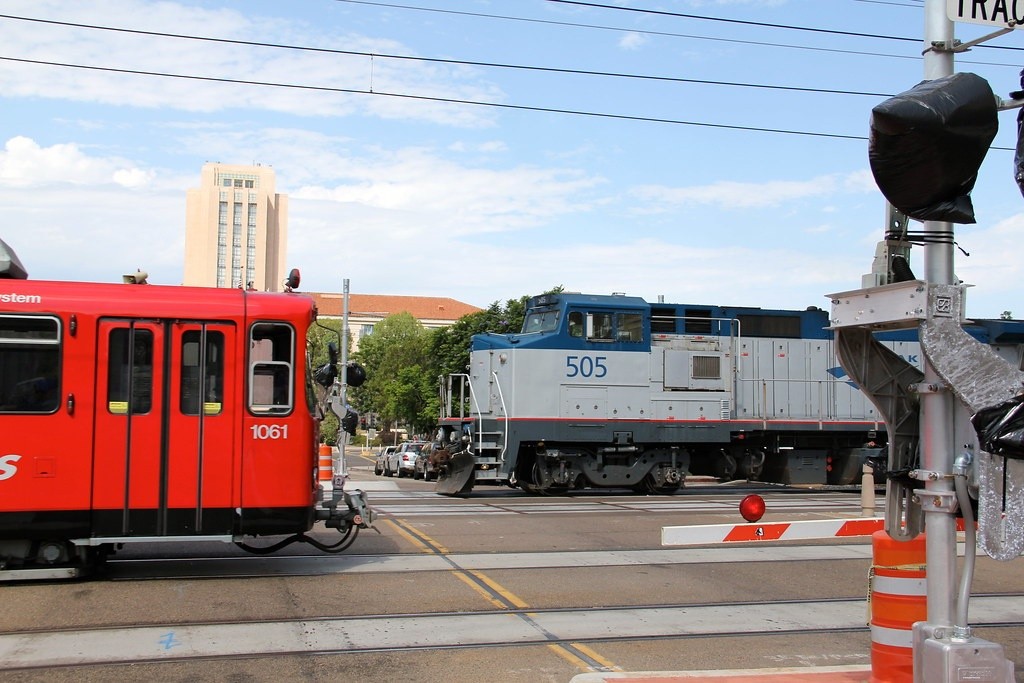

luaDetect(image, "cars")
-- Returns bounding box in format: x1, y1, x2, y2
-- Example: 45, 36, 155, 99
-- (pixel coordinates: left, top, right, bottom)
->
376, 445, 402, 476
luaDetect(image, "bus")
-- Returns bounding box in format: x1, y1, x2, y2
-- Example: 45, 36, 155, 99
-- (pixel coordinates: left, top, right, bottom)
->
0, 236, 380, 586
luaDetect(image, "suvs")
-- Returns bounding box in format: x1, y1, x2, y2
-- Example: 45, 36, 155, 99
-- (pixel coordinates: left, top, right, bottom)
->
386, 439, 436, 479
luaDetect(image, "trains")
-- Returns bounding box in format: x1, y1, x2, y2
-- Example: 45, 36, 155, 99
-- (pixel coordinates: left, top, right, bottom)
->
433, 288, 1024, 498
